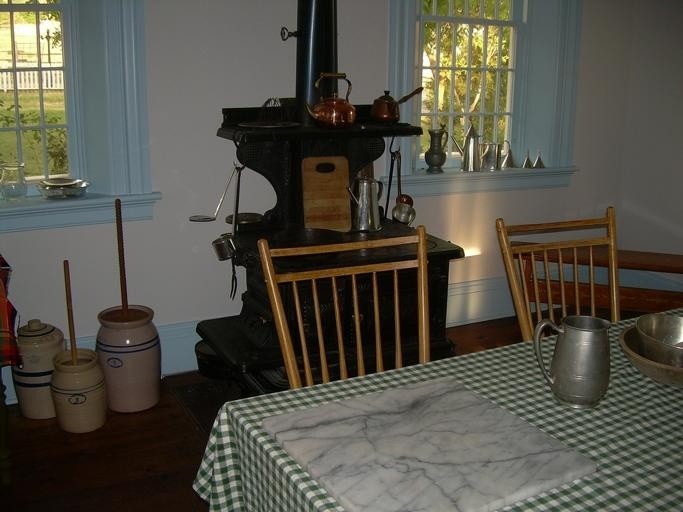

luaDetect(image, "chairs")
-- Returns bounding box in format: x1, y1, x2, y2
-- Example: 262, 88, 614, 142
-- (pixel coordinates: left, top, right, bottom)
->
496, 206, 620, 342
258, 225, 431, 388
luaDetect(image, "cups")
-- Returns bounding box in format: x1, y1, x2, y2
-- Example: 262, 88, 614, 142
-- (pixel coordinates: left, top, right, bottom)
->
391, 203, 416, 225
478, 142, 497, 170
211, 235, 239, 261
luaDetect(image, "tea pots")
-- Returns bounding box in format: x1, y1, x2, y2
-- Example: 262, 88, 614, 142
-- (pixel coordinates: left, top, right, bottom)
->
424, 124, 448, 173
450, 125, 489, 171
534, 315, 612, 409
302, 71, 355, 127
346, 175, 382, 234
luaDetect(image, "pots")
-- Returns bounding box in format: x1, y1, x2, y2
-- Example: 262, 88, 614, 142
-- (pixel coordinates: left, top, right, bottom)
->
370, 86, 423, 125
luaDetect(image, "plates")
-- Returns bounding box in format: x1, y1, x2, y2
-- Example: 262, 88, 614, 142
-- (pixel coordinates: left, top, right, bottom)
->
239, 122, 300, 129
37, 179, 91, 200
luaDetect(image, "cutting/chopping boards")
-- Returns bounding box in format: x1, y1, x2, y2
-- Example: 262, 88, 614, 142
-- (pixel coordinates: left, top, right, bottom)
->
299, 156, 350, 232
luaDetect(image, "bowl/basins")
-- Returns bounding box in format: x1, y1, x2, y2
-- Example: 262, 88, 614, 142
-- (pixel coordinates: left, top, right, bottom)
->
637, 314, 682, 367
618, 324, 682, 385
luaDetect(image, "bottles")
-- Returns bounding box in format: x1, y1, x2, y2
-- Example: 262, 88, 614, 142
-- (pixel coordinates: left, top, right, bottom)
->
0, 160, 27, 202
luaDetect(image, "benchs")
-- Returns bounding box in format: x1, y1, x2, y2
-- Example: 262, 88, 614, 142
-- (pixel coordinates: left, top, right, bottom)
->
511, 241, 683, 322
